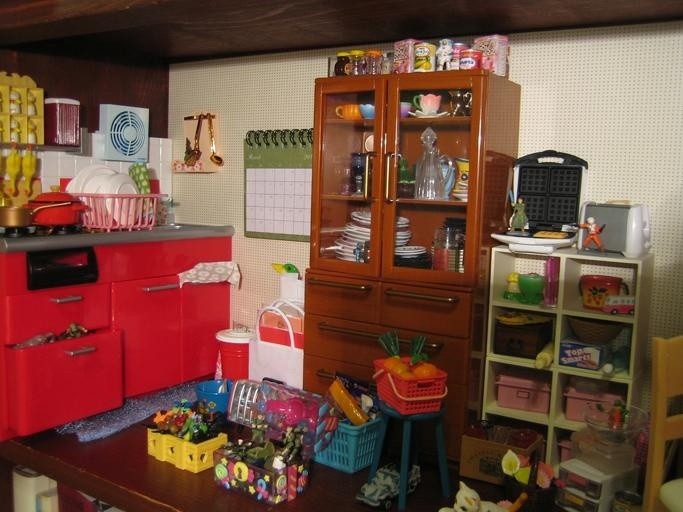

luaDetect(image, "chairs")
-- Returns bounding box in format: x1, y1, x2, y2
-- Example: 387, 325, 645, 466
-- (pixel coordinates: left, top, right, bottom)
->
640, 331, 683, 511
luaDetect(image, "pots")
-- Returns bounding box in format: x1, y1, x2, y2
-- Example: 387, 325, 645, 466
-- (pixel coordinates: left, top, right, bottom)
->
28, 185, 89, 227
0, 201, 74, 228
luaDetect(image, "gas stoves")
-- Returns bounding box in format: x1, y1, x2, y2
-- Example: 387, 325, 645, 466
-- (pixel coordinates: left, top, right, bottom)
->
0, 222, 90, 250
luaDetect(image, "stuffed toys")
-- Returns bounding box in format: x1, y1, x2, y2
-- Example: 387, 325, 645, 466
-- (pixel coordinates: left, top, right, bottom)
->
439, 480, 508, 512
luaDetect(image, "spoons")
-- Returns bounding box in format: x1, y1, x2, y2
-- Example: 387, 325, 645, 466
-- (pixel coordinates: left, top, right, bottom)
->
206, 115, 223, 165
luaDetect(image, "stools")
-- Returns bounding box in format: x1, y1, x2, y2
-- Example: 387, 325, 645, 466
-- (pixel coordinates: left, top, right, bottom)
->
368, 398, 453, 512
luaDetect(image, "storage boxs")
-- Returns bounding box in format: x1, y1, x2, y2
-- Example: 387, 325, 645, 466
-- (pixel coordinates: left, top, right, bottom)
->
44, 97, 81, 147
459, 421, 545, 487
496, 370, 550, 416
563, 383, 624, 423
314, 409, 389, 473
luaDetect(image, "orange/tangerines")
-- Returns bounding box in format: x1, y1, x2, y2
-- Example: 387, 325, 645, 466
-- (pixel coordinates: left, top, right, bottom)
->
384, 358, 398, 370
416, 363, 438, 377
392, 364, 409, 374
401, 372, 414, 379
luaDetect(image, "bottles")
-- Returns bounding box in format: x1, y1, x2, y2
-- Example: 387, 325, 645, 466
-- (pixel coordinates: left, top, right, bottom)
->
351, 50, 366, 76
352, 152, 367, 195
338, 167, 352, 196
335, 51, 352, 76
431, 227, 459, 272
364, 49, 382, 74
381, 52, 393, 74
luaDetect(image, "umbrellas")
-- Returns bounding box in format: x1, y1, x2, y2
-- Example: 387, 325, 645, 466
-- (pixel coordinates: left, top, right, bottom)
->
318, 366, 376, 397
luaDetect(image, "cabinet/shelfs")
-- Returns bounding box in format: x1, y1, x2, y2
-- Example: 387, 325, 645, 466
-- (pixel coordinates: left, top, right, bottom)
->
300, 68, 521, 462
480, 241, 654, 474
0, 222, 234, 442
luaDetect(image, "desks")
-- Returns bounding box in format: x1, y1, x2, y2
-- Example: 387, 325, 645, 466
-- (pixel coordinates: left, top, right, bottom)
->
0, 408, 380, 512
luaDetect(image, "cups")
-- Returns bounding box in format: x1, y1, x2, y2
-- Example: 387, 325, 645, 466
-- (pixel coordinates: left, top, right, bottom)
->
414, 93, 442, 114
362, 104, 375, 117
399, 101, 414, 117
335, 105, 361, 119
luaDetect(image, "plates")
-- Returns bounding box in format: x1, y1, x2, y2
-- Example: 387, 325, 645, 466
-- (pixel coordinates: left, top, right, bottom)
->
334, 211, 426, 263
432, 245, 464, 273
452, 181, 468, 199
408, 112, 448, 118
64, 163, 141, 227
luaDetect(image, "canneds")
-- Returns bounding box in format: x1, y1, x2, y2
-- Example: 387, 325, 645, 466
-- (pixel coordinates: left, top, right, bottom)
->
460, 49, 481, 70
452, 42, 469, 70
414, 42, 437, 73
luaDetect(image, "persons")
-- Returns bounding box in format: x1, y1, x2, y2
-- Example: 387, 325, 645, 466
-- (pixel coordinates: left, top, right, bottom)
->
508, 191, 529, 236
572, 217, 606, 253
